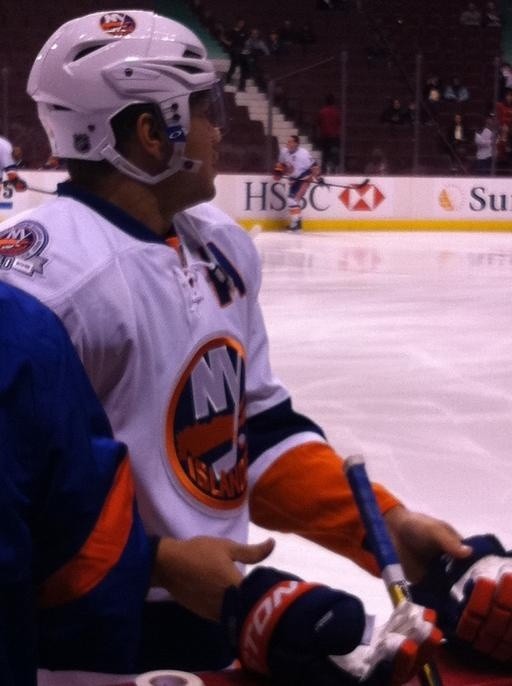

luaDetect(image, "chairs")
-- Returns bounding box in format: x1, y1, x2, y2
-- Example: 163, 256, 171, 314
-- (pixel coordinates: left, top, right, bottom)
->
0, 1, 280, 174
178, 0, 511, 177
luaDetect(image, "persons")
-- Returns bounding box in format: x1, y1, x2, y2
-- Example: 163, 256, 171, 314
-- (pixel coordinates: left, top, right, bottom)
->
225, 1, 511, 173
1, 131, 66, 223
273, 135, 325, 230
0, 10, 474, 685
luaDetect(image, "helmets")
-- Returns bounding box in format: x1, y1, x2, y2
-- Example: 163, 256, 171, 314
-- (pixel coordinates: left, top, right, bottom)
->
28, 11, 220, 183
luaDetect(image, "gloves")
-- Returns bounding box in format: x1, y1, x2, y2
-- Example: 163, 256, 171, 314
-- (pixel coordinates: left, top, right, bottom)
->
219, 565, 443, 684
414, 535, 512, 670
